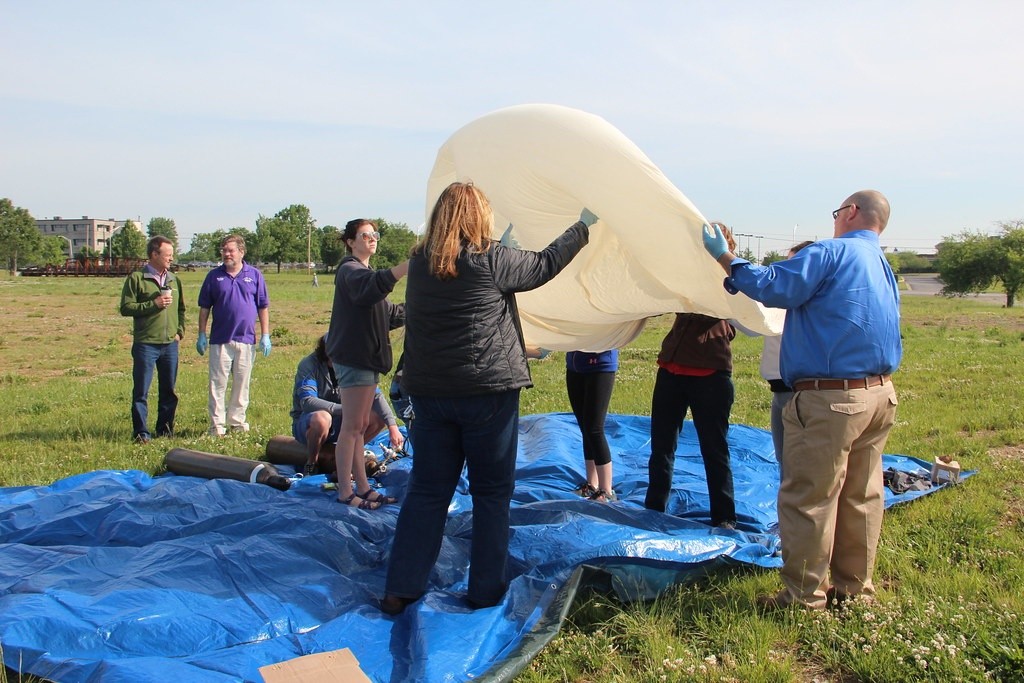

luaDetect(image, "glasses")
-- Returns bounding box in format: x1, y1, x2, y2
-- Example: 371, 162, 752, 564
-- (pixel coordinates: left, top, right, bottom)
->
219, 249, 235, 254
357, 232, 381, 241
832, 205, 860, 220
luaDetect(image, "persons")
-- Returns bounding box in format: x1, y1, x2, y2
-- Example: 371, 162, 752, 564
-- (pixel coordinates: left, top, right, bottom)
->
729, 240, 815, 483
324, 218, 410, 509
312, 273, 318, 288
702, 190, 902, 615
526, 348, 618, 503
197, 234, 272, 436
291, 331, 404, 477
644, 222, 737, 530
382, 183, 599, 616
120, 236, 186, 445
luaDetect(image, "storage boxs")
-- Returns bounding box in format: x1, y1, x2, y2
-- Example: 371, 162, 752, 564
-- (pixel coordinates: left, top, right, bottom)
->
931, 456, 960, 484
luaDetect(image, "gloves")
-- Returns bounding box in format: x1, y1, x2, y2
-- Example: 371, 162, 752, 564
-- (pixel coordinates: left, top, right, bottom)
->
580, 208, 598, 227
259, 334, 273, 357
500, 224, 520, 251
197, 333, 208, 355
702, 224, 728, 261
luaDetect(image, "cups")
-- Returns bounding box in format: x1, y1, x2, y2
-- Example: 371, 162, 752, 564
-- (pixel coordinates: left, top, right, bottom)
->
161, 285, 172, 296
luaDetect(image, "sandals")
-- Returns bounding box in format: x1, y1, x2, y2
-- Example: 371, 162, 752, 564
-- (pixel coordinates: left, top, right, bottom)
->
357, 487, 397, 504
338, 495, 380, 510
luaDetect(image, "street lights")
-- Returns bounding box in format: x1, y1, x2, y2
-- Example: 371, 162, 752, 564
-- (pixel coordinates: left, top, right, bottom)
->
109, 226, 125, 265
58, 235, 73, 267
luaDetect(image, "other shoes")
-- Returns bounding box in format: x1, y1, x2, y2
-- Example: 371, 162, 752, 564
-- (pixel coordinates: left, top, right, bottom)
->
717, 521, 734, 530
135, 437, 150, 446
756, 596, 777, 612
303, 462, 319, 477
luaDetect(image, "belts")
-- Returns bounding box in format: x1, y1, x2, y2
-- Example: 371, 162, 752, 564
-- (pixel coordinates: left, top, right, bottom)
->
792, 374, 891, 392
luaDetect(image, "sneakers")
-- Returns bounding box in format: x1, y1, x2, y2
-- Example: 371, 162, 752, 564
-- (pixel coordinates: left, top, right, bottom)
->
572, 482, 602, 500
589, 490, 618, 505
